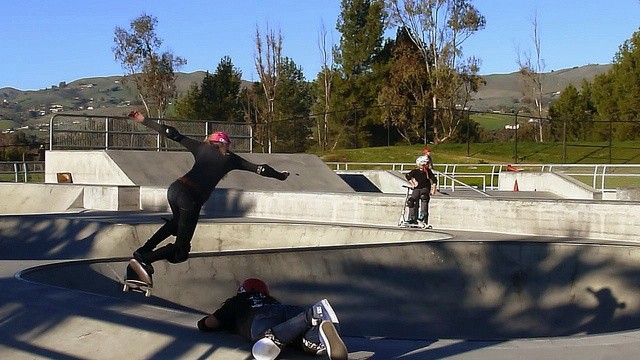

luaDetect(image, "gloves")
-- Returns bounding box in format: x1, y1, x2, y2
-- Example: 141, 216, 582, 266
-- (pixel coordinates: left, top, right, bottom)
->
409, 178, 418, 188
430, 183, 436, 196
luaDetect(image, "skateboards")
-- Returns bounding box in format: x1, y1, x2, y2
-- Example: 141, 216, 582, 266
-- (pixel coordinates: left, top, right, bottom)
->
123, 279, 152, 297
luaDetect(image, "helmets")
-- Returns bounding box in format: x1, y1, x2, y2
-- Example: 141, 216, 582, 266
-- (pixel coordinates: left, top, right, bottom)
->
424, 148, 431, 153
209, 131, 231, 145
237, 278, 269, 297
416, 156, 430, 166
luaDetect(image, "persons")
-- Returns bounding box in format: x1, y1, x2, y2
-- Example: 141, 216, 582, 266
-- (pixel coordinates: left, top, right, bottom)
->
407, 155, 437, 228
197, 278, 349, 359
422, 148, 433, 171
123, 110, 290, 288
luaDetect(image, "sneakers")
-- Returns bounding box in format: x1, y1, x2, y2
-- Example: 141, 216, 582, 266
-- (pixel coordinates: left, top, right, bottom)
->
317, 320, 349, 360
130, 255, 154, 285
311, 298, 344, 337
417, 218, 428, 225
126, 264, 138, 280
404, 218, 418, 224
